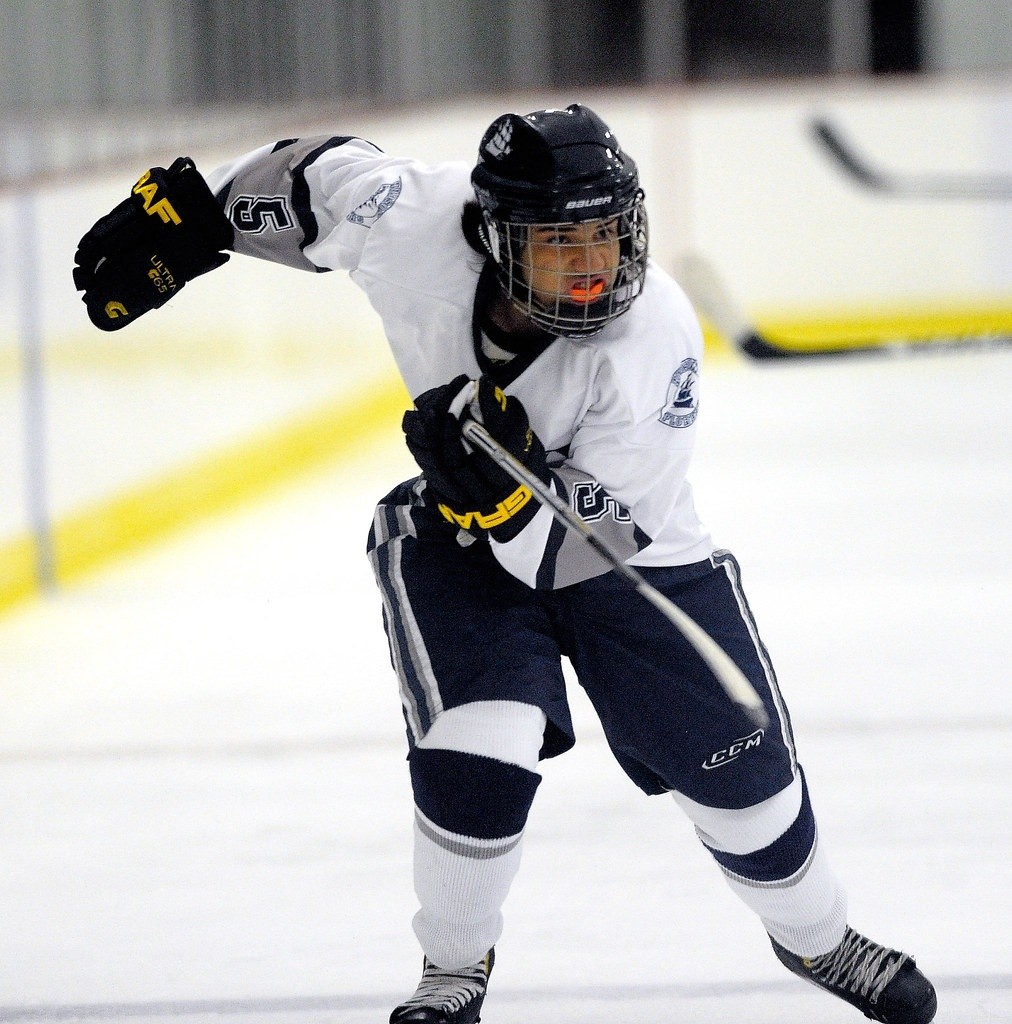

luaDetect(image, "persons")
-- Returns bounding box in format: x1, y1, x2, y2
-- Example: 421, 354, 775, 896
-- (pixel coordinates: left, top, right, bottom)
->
73, 104, 938, 1023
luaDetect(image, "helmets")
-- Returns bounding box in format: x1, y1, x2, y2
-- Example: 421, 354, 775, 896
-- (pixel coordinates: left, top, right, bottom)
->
469, 103, 650, 341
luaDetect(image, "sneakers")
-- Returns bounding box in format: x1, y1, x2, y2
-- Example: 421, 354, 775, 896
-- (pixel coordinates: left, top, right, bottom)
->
389, 945, 494, 1024
769, 926, 937, 1024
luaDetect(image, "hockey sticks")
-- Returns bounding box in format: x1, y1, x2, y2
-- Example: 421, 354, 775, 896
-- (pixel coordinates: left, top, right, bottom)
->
458, 413, 771, 732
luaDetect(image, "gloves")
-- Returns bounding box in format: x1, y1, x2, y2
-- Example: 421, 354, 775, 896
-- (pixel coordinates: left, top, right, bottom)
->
73, 157, 233, 332
402, 372, 548, 534
